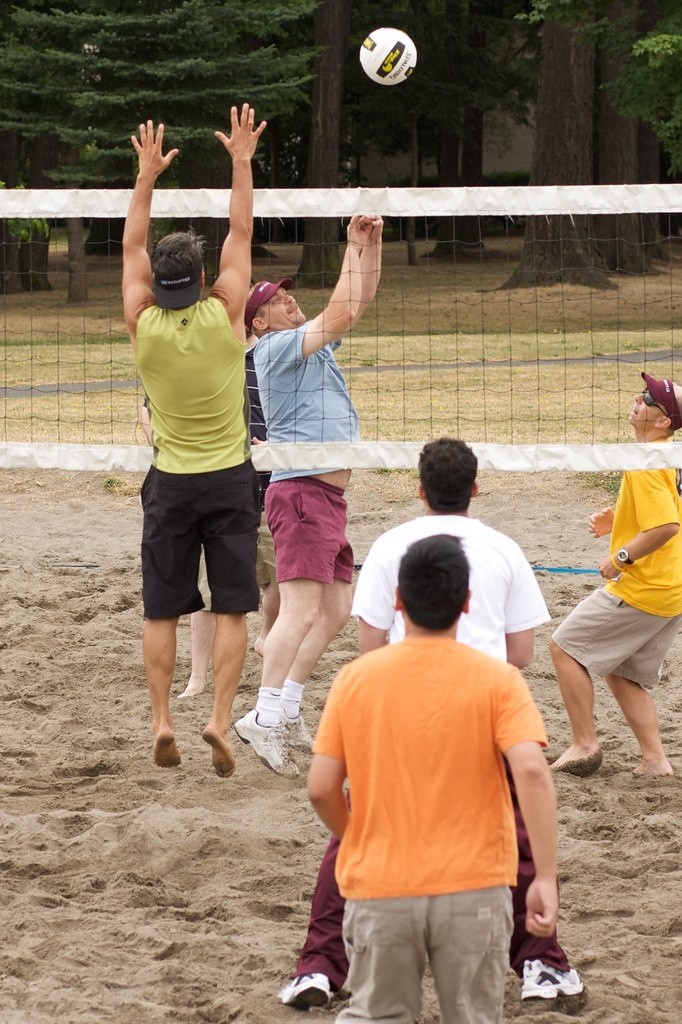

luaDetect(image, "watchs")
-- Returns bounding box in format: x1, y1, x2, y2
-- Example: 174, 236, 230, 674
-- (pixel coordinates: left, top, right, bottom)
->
618, 546, 634, 565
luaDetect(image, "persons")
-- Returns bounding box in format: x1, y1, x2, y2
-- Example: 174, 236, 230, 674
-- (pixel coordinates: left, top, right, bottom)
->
277, 437, 584, 1010
307, 534, 559, 1024
141, 278, 281, 698
123, 103, 267, 778
235, 215, 384, 778
548, 372, 682, 774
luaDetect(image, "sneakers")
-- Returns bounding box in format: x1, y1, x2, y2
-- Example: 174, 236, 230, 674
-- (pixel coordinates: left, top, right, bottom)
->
276, 707, 315, 756
271, 972, 333, 1013
231, 709, 302, 782
520, 958, 584, 1002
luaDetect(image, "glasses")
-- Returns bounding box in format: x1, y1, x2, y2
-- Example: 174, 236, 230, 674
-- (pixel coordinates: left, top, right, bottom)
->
641, 386, 669, 418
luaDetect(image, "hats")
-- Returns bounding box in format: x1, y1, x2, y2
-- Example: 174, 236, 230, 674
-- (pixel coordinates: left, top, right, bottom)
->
641, 370, 682, 430
154, 263, 204, 310
243, 277, 292, 331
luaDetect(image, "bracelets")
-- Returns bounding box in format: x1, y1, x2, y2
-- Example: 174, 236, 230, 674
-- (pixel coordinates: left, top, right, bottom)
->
611, 553, 626, 572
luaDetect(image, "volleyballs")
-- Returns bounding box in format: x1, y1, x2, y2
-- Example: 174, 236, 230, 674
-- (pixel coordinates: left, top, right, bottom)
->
358, 26, 418, 86
